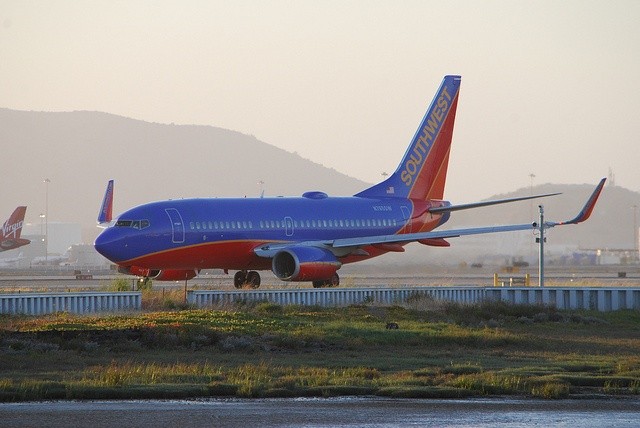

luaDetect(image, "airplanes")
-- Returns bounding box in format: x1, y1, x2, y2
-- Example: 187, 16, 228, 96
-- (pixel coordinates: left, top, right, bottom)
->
95, 76, 606, 289
0, 207, 30, 251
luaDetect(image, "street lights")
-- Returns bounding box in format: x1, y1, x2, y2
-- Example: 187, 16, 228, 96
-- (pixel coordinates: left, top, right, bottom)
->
43, 178, 48, 274
39, 214, 45, 242
